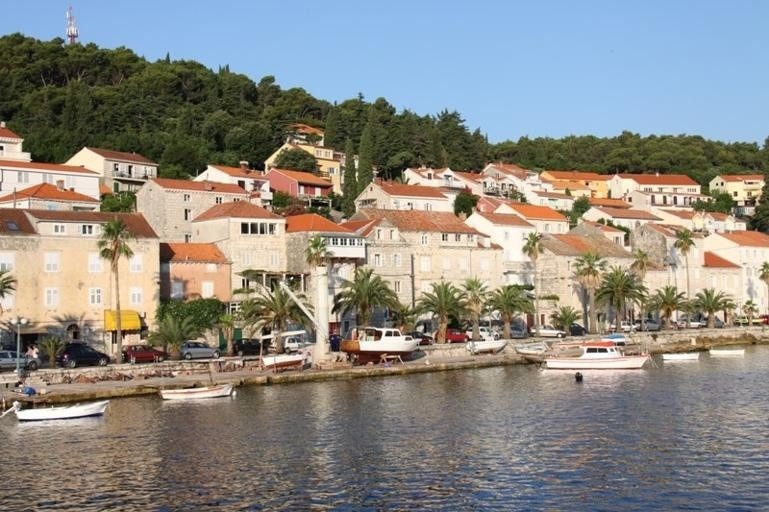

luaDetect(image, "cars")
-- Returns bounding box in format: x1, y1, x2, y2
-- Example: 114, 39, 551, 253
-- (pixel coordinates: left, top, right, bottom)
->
635, 319, 660, 332
463, 326, 500, 340
434, 328, 469, 343
55, 342, 109, 369
569, 322, 586, 337
120, 345, 170, 363
676, 312, 707, 328
530, 322, 566, 339
328, 333, 344, 352
702, 317, 725, 329
501, 324, 530, 338
406, 333, 430, 345
608, 320, 640, 332
267, 336, 311, 354
230, 339, 268, 357
733, 315, 767, 326
0, 349, 41, 372
180, 340, 221, 360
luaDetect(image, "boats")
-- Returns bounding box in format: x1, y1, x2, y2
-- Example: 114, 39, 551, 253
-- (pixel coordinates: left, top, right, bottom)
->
339, 324, 423, 355
466, 341, 506, 353
513, 335, 638, 363
261, 350, 307, 371
16, 400, 107, 419
659, 352, 698, 360
156, 382, 233, 400
708, 347, 744, 355
542, 354, 649, 370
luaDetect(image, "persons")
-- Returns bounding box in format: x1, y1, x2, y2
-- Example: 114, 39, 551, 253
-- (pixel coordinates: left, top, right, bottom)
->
24, 345, 34, 357
32, 345, 40, 359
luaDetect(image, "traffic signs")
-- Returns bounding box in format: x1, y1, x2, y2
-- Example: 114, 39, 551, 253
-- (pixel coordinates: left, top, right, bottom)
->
11, 315, 27, 372
